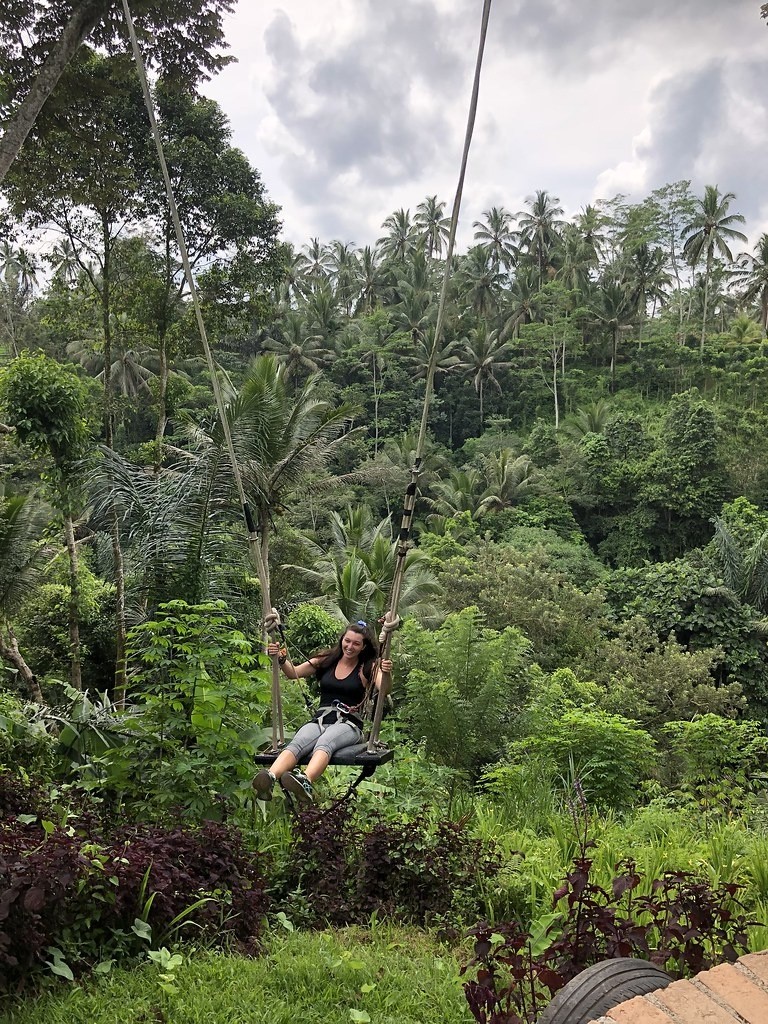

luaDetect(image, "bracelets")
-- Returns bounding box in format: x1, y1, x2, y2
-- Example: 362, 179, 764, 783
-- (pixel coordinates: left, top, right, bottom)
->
279, 649, 286, 664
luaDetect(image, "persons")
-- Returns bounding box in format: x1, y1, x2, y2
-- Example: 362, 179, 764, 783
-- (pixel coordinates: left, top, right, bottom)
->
252, 621, 393, 804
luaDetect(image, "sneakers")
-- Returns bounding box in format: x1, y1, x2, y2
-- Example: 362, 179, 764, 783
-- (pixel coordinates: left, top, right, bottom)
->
253, 769, 275, 802
281, 768, 315, 805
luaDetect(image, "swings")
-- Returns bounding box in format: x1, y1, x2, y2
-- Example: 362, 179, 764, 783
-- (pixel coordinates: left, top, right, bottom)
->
118, 0, 495, 769
